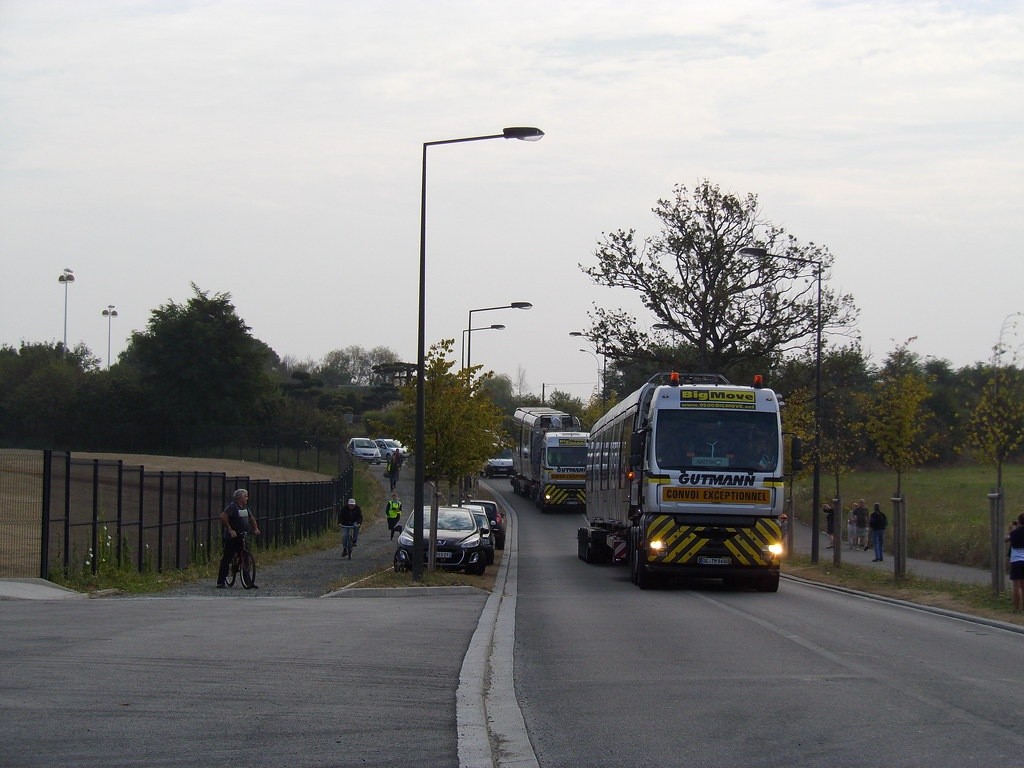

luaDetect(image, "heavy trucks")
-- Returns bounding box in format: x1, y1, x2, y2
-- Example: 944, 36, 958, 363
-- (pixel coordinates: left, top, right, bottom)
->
509, 407, 591, 513
576, 372, 803, 593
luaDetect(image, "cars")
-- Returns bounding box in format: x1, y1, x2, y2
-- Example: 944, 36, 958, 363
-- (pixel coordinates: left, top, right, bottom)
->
393, 506, 489, 577
346, 438, 381, 465
450, 504, 496, 565
462, 500, 506, 550
374, 439, 407, 461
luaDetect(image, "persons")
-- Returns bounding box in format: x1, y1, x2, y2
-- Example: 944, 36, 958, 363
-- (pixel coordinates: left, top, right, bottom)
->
869, 504, 888, 561
1004, 512, 1024, 611
780, 514, 787, 549
848, 499, 869, 551
387, 449, 403, 491
217, 488, 260, 588
822, 505, 834, 548
386, 493, 402, 540
338, 499, 363, 557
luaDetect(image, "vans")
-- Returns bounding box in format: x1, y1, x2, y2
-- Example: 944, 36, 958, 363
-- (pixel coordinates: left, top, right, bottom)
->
478, 444, 514, 479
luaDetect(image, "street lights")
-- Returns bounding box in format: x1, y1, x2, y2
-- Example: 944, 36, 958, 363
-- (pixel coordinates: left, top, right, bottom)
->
411, 126, 546, 580
58, 268, 76, 374
465, 301, 534, 505
460, 324, 506, 387
652, 322, 708, 373
580, 349, 600, 420
568, 331, 606, 416
101, 304, 119, 371
739, 248, 822, 566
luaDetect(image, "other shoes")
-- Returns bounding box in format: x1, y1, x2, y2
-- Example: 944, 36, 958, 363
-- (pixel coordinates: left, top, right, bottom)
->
354, 541, 358, 546
217, 583, 226, 589
826, 545, 833, 549
864, 546, 868, 551
342, 550, 347, 557
245, 585, 258, 589
856, 546, 860, 551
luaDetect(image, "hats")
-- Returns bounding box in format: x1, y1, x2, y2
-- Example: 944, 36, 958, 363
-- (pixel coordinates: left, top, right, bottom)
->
348, 499, 356, 505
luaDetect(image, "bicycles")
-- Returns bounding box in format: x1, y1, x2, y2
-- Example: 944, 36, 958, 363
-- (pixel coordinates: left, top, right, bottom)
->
340, 525, 362, 561
220, 530, 262, 590
